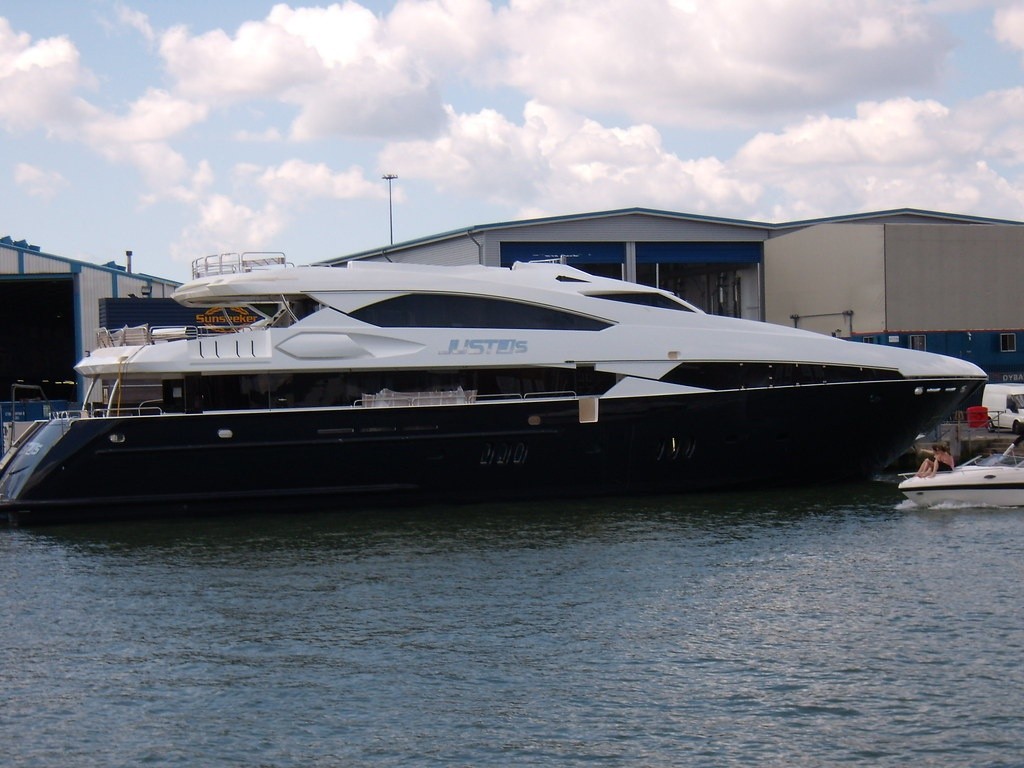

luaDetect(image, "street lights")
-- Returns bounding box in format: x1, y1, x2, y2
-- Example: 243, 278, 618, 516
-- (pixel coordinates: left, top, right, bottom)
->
381, 173, 399, 245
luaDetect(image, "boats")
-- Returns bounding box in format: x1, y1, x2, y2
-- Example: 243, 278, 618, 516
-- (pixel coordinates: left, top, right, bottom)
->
897, 435, 1024, 507
0, 249, 989, 516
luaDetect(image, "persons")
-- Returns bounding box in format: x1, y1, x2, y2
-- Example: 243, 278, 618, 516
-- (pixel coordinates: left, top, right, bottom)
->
916, 440, 956, 477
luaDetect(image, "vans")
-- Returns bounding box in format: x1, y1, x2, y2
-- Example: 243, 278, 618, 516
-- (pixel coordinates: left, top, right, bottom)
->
983, 383, 1024, 432
